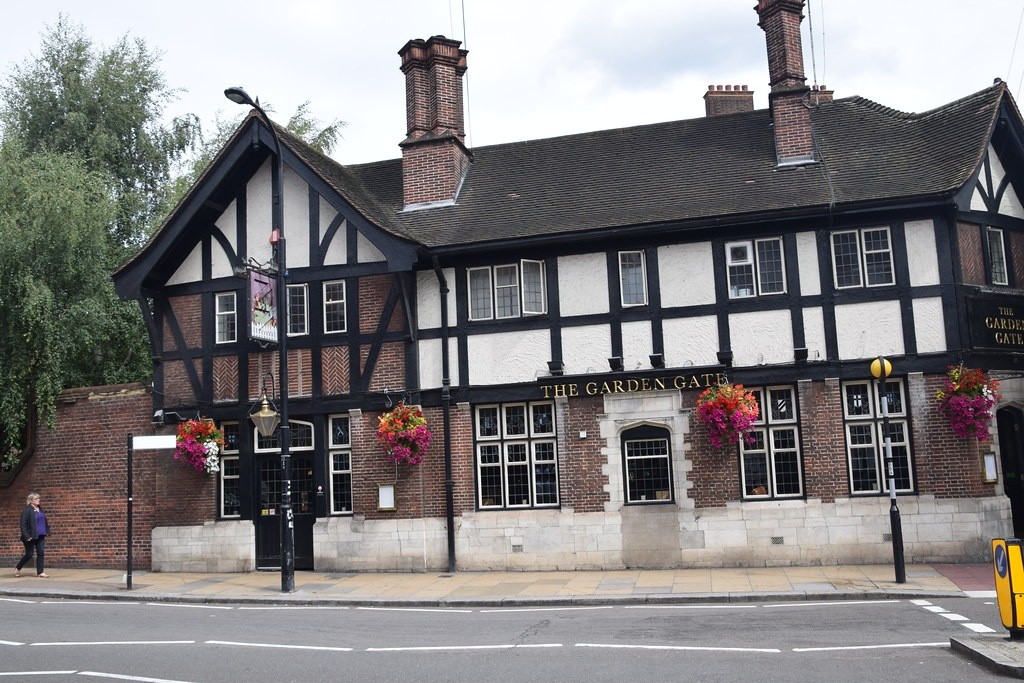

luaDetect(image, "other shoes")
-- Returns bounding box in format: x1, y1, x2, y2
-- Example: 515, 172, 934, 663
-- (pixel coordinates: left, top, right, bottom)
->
38, 573, 50, 578
14, 565, 20, 577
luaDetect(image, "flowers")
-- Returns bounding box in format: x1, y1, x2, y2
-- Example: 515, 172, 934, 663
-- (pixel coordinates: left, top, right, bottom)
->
935, 365, 1003, 442
173, 415, 228, 475
376, 400, 432, 467
696, 383, 760, 449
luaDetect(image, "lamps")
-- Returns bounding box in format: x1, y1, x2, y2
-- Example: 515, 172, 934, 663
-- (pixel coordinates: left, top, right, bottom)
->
793, 348, 808, 364
547, 360, 565, 376
649, 353, 665, 369
246, 370, 281, 440
716, 351, 734, 367
608, 356, 624, 372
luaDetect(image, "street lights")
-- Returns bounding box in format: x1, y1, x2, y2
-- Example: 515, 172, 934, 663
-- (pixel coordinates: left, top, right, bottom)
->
870, 355, 909, 584
221, 85, 297, 592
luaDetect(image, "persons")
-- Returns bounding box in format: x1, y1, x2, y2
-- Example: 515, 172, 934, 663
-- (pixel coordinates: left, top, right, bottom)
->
14, 492, 52, 578
